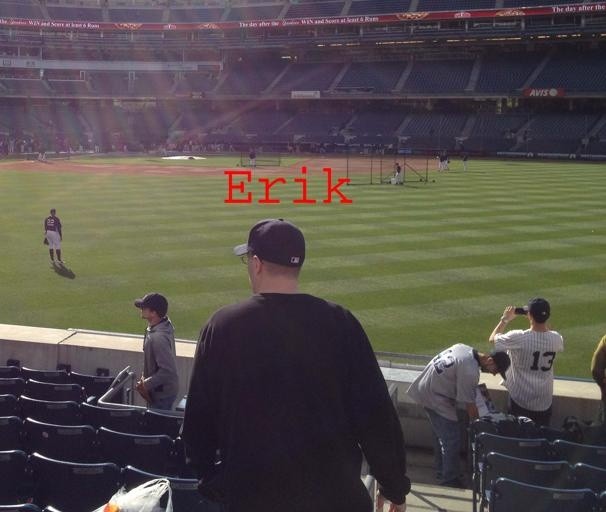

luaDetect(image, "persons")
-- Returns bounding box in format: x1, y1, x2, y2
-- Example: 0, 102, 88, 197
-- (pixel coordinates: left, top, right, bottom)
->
489, 297, 564, 426
249, 149, 256, 168
434, 148, 468, 172
404, 343, 510, 486
183, 219, 411, 512
44, 209, 63, 264
590, 335, 606, 424
133, 293, 179, 410
395, 163, 403, 184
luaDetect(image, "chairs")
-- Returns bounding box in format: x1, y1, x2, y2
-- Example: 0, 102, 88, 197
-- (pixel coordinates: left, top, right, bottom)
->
124, 465, 204, 512
0, 450, 28, 503
68, 373, 127, 408
571, 461, 606, 494
18, 395, 80, 427
0, 414, 25, 451
81, 400, 144, 435
21, 367, 67, 384
145, 407, 184, 440
69, 369, 124, 404
0, 376, 27, 394
28, 450, 119, 512
97, 426, 176, 478
487, 476, 597, 512
0, 502, 40, 512
550, 439, 605, 469
0, 365, 21, 378
480, 450, 572, 512
472, 430, 553, 512
0, 393, 20, 416
23, 416, 98, 464
26, 379, 96, 403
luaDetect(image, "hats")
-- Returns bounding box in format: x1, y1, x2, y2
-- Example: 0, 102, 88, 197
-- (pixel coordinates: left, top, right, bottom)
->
231, 217, 307, 268
132, 292, 169, 316
489, 350, 513, 382
523, 296, 552, 322
